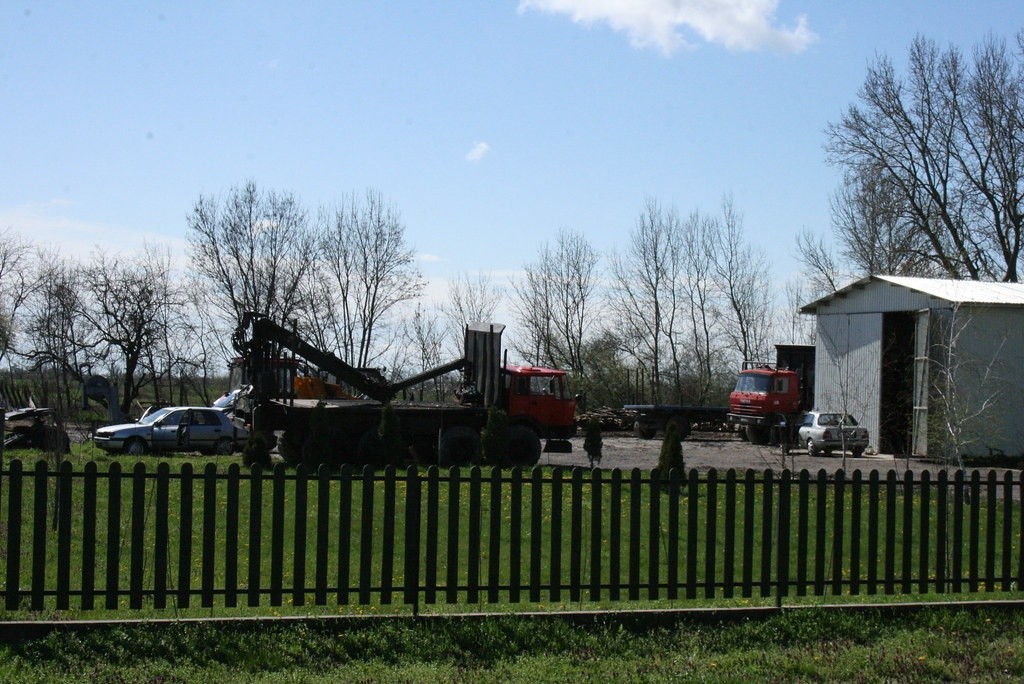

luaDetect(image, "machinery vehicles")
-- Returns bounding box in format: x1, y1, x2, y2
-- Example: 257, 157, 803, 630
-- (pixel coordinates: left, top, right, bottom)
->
225, 353, 396, 403
223, 310, 580, 467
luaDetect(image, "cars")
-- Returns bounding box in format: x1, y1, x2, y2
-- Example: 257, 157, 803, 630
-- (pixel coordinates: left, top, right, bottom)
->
93, 405, 235, 457
211, 386, 252, 448
771, 410, 869, 457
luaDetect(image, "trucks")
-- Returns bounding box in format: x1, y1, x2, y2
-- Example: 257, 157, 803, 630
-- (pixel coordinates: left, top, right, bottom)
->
622, 402, 730, 439
723, 343, 817, 445
